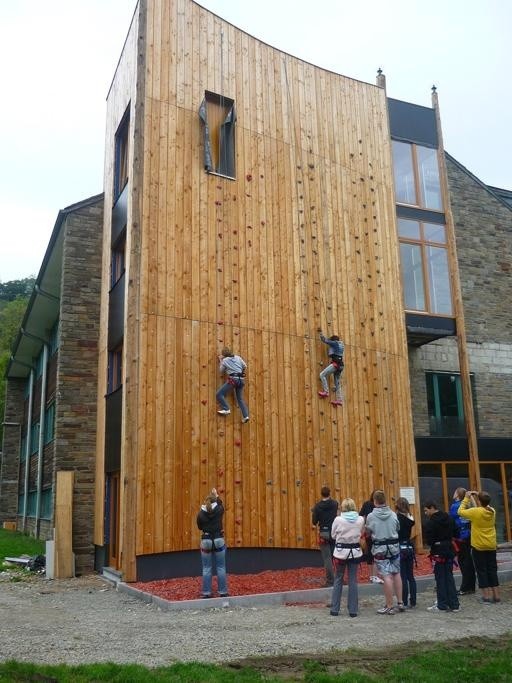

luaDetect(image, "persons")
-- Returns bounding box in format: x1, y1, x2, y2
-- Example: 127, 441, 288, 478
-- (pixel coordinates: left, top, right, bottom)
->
316, 331, 346, 406
216, 347, 250, 423
195, 486, 231, 598
311, 486, 501, 617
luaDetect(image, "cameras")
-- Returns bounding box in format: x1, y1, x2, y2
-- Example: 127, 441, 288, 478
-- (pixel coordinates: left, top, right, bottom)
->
470, 493, 477, 496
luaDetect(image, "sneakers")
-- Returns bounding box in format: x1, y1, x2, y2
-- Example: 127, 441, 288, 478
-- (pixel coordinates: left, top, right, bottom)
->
494, 595, 502, 604
318, 391, 329, 398
426, 603, 448, 614
479, 596, 491, 605
216, 408, 231, 415
321, 580, 333, 587
377, 602, 406, 615
331, 399, 343, 406
455, 589, 474, 596
241, 416, 249, 424
349, 611, 357, 618
328, 608, 338, 616
